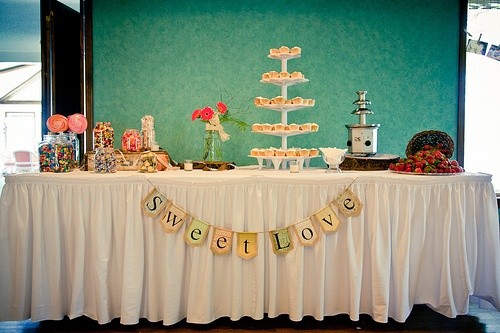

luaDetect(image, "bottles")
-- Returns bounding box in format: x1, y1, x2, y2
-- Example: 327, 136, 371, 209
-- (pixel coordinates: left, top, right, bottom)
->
139, 115, 155, 150
93, 122, 115, 151
94, 147, 116, 173
38, 133, 79, 173
121, 129, 142, 151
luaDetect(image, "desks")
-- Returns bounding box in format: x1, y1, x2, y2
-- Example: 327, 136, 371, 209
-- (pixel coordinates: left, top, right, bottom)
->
0, 164, 500, 333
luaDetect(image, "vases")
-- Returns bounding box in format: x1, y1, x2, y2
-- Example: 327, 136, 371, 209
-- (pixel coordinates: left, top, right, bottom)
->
202, 130, 223, 162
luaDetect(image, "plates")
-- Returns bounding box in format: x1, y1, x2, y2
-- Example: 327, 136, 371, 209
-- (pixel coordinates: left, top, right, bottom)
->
390, 168, 461, 176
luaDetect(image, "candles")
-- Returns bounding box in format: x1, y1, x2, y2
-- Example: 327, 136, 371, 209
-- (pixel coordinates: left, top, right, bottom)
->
289, 161, 300, 173
150, 141, 160, 152
184, 159, 194, 171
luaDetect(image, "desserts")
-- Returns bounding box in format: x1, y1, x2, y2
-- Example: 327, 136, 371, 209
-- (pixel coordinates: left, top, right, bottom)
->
261, 70, 304, 79
251, 122, 319, 132
250, 146, 318, 157
254, 96, 316, 106
269, 45, 301, 55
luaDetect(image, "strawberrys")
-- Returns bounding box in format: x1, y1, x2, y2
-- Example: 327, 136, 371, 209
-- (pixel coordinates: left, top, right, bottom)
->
389, 145, 462, 174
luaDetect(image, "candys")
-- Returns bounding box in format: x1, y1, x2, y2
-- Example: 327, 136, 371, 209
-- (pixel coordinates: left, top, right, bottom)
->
37, 114, 87, 173
122, 130, 142, 152
93, 121, 117, 173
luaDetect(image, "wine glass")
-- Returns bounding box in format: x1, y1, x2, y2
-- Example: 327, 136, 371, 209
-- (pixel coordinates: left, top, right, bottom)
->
319, 148, 348, 173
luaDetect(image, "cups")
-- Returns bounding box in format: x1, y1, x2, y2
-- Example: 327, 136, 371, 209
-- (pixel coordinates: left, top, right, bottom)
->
184, 160, 193, 170
151, 141, 159, 151
290, 161, 299, 173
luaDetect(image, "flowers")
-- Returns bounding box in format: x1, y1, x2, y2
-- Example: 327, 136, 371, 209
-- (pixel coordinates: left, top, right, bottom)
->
191, 87, 254, 161
